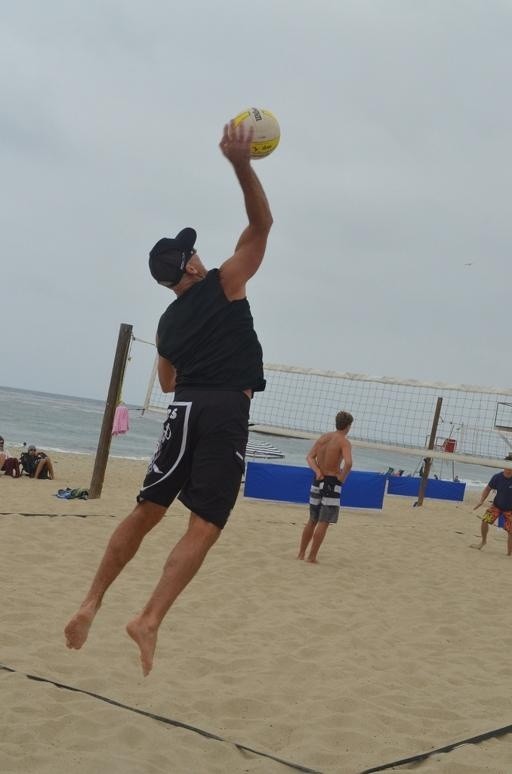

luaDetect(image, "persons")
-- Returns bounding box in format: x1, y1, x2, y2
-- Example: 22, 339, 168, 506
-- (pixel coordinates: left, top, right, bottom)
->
474, 456, 512, 555
0, 438, 20, 478
298, 411, 354, 564
420, 462, 425, 476
21, 445, 54, 479
65, 118, 275, 676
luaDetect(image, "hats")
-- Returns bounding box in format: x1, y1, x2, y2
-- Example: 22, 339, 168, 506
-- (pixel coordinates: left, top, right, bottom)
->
148, 227, 196, 288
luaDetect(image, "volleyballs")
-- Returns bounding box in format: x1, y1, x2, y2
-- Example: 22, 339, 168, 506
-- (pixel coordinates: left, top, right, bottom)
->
232, 107, 281, 159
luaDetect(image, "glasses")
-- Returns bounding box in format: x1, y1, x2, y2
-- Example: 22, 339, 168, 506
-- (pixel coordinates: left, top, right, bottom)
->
30, 449, 36, 451
